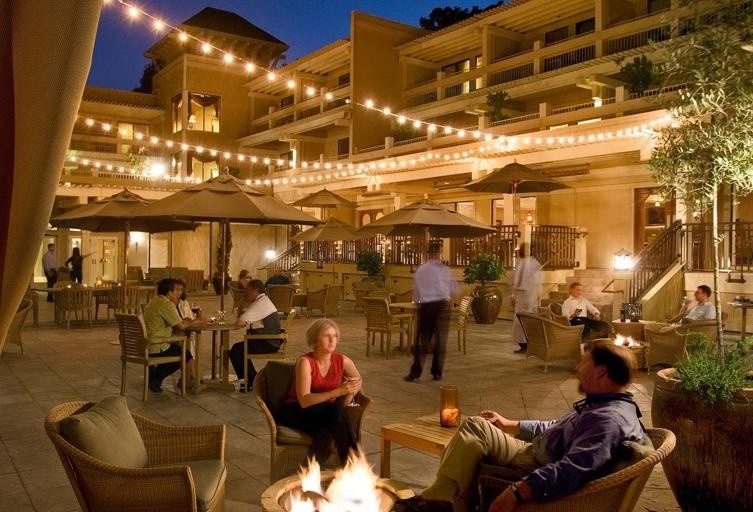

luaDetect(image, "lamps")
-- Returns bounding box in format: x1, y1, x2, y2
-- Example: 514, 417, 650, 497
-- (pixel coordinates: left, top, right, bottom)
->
612, 246, 630, 271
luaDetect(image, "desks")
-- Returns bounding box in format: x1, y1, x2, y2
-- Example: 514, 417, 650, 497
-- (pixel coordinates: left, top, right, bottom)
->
614, 319, 652, 340
183, 320, 246, 394
378, 412, 472, 483
726, 302, 752, 341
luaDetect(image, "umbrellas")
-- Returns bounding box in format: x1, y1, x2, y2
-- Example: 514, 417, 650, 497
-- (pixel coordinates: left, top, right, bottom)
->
460, 157, 571, 224
355, 194, 500, 263
47, 188, 201, 311
287, 187, 360, 221
291, 218, 358, 286
121, 166, 326, 312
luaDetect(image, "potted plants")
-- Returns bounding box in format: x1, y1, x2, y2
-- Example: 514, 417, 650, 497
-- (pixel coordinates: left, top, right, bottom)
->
212, 222, 235, 294
459, 249, 504, 324
649, 0, 753, 511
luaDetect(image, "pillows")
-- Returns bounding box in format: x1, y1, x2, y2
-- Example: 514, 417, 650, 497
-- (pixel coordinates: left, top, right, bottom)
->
604, 435, 653, 474
265, 360, 298, 417
60, 397, 149, 471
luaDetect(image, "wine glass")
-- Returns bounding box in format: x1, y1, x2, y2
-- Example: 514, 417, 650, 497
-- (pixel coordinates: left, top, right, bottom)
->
192, 302, 200, 319
345, 376, 361, 407
576, 306, 582, 320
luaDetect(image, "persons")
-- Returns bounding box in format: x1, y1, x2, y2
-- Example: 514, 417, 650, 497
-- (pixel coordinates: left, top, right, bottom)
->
560, 282, 609, 340
229, 279, 281, 390
65, 248, 97, 284
404, 243, 462, 382
677, 284, 717, 325
510, 242, 545, 353
175, 279, 196, 322
42, 243, 57, 303
142, 279, 192, 393
390, 339, 649, 512
278, 318, 364, 471
264, 266, 289, 287
239, 270, 252, 289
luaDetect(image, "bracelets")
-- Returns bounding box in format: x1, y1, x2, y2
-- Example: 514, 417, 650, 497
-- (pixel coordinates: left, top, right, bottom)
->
507, 480, 525, 506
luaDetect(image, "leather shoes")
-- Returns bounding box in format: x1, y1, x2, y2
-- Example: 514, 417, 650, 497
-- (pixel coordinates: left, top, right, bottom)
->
514, 348, 527, 354
239, 384, 256, 393
393, 495, 454, 512
434, 374, 441, 380
403, 374, 419, 382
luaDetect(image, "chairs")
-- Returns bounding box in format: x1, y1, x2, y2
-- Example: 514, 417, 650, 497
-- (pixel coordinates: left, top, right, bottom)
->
252, 358, 374, 486
53, 281, 77, 287
515, 311, 584, 373
114, 312, 186, 402
477, 427, 677, 512
646, 316, 723, 370
44, 400, 227, 512
244, 309, 296, 392
546, 299, 570, 324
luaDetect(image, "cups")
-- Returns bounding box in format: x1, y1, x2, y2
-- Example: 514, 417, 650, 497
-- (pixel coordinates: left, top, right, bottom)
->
479, 391, 495, 419
439, 384, 461, 427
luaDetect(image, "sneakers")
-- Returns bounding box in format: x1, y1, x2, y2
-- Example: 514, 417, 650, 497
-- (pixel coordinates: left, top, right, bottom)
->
149, 382, 163, 393
